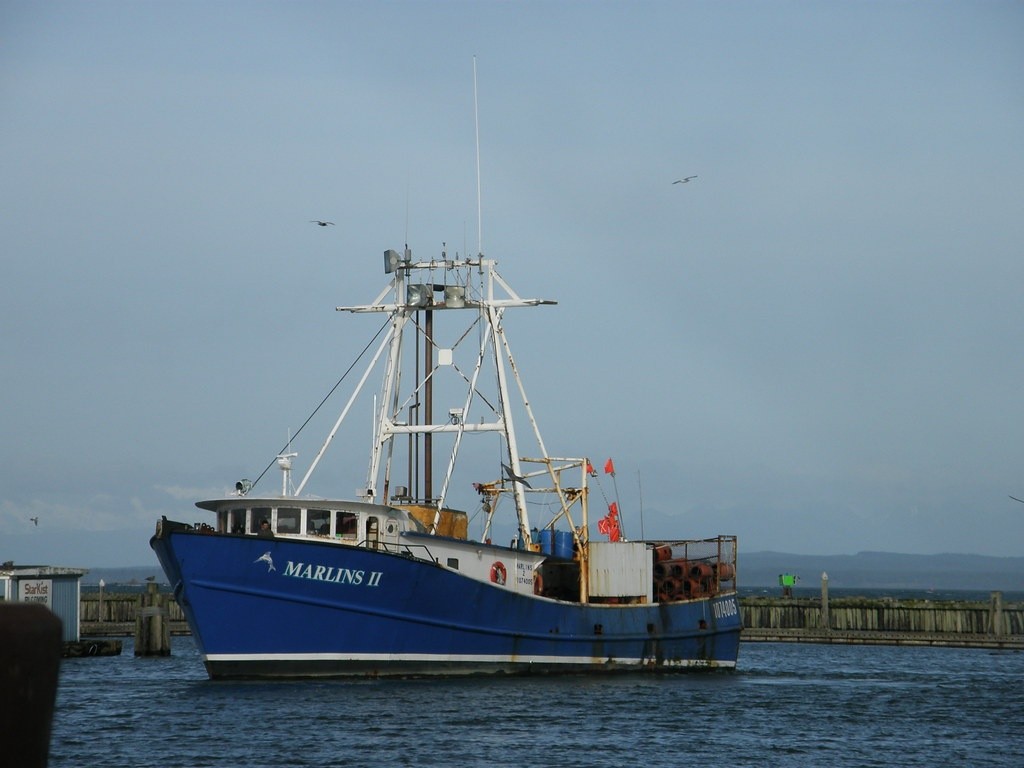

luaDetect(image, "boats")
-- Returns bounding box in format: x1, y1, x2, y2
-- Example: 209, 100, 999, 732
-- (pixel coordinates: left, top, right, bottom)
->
149, 54, 744, 682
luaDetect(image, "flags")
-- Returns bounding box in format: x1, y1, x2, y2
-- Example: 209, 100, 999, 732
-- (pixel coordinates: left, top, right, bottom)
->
598, 502, 620, 541
605, 459, 613, 472
586, 463, 592, 473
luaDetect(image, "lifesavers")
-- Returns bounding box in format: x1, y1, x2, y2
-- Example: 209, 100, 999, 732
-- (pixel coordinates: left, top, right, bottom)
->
533, 571, 544, 595
490, 561, 507, 585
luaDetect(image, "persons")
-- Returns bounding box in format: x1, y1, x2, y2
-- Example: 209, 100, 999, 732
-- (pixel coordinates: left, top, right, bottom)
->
258, 518, 274, 536
495, 566, 504, 584
